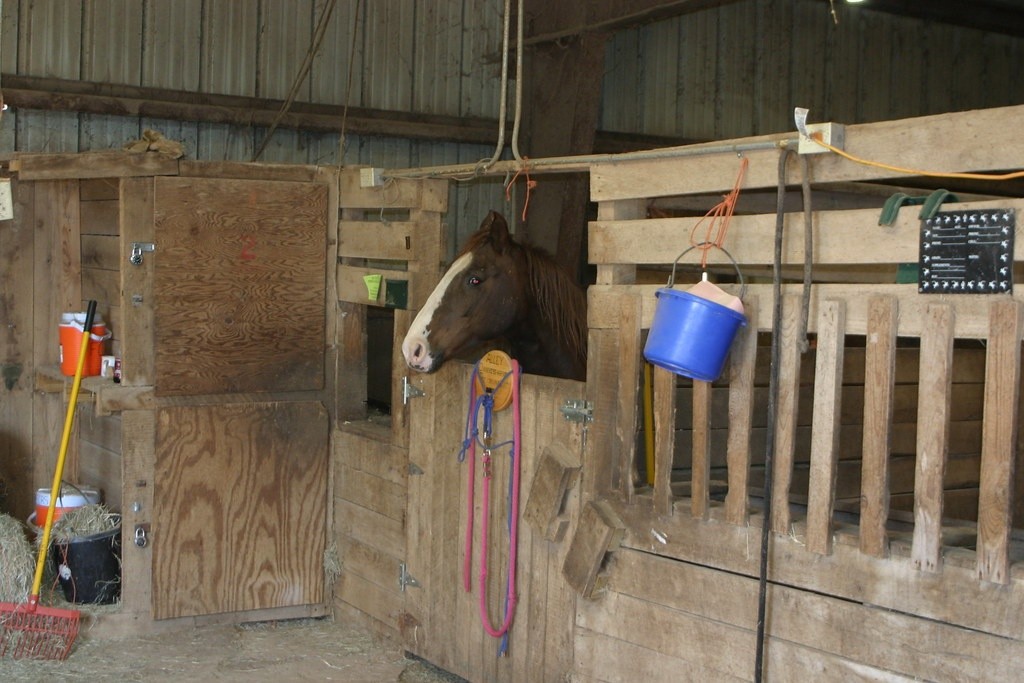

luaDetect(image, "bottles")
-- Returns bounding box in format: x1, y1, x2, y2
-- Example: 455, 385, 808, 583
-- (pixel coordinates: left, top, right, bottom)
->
113, 341, 122, 383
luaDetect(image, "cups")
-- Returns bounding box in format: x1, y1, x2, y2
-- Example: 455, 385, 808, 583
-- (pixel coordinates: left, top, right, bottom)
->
100, 355, 115, 379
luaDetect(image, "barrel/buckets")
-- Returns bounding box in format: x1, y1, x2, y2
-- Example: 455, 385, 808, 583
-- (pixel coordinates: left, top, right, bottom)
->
643, 242, 748, 383
59, 313, 112, 379
50, 480, 121, 605
27, 486, 101, 574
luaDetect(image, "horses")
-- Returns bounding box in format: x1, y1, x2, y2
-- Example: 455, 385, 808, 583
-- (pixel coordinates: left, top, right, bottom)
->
401, 210, 588, 380
